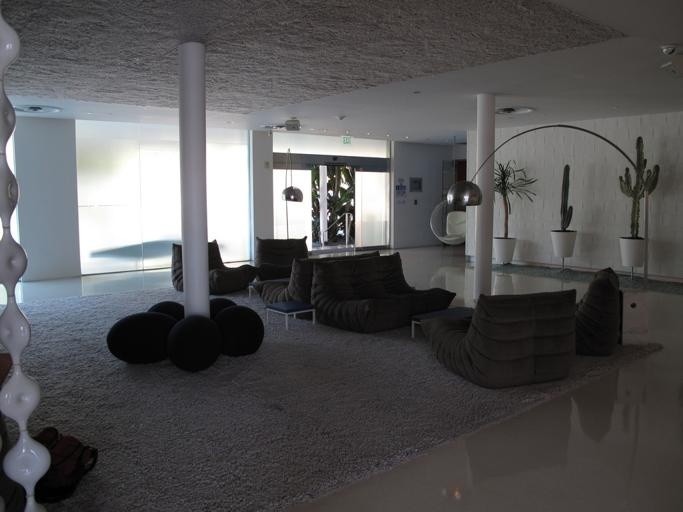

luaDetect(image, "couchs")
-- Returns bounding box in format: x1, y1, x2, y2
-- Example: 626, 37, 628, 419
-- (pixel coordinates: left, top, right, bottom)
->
260, 251, 380, 319
310, 252, 456, 333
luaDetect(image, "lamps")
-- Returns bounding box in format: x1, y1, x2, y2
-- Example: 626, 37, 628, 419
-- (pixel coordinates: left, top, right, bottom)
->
447, 124, 650, 280
282, 149, 303, 202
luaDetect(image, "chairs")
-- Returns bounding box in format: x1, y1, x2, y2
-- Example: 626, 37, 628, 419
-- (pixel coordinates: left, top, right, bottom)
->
256, 236, 309, 281
429, 289, 577, 390
170, 240, 259, 295
576, 267, 619, 357
430, 199, 465, 246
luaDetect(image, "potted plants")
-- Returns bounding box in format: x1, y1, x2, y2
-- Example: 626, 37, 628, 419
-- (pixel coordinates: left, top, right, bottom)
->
550, 164, 577, 258
619, 137, 660, 267
479, 159, 538, 264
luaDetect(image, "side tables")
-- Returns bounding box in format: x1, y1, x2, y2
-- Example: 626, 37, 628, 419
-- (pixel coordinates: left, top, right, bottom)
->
264, 301, 317, 331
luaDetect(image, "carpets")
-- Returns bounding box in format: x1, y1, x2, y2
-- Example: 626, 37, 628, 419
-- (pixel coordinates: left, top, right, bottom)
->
1, 287, 666, 512
441, 254, 683, 295
